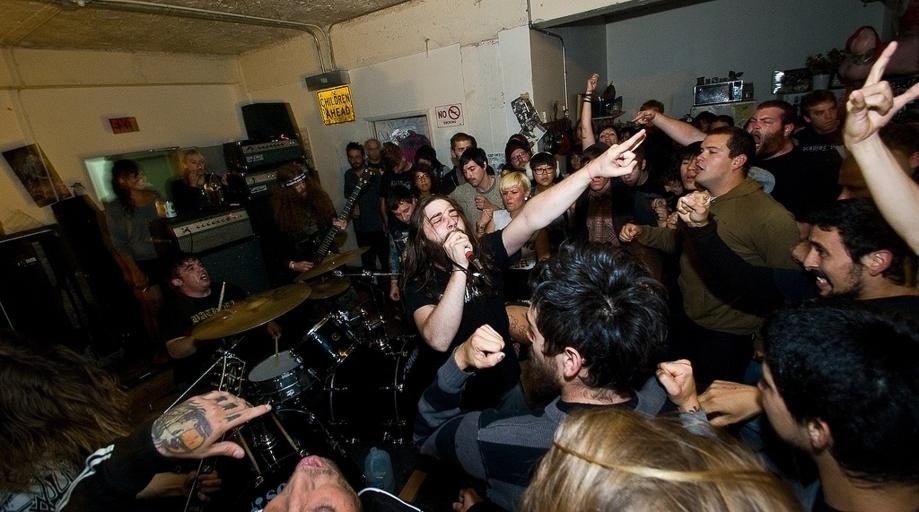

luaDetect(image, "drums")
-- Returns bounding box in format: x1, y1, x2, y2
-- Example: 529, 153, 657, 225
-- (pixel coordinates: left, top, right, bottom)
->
288, 312, 362, 378
368, 318, 393, 353
248, 351, 315, 403
257, 432, 285, 468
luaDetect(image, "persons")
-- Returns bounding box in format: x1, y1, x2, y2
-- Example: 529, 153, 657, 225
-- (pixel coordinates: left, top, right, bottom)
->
2, 3, 918, 511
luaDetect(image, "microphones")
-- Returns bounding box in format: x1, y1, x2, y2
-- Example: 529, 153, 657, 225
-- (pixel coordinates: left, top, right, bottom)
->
448, 229, 488, 277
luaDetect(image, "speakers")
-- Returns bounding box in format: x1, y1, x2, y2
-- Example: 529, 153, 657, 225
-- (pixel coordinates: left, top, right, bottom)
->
243, 103, 295, 140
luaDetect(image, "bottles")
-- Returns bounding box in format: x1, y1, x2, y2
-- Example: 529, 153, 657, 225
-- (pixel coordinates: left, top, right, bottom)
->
155, 199, 177, 218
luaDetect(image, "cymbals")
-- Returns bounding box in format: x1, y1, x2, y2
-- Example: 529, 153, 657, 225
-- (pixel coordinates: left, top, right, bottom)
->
310, 283, 347, 300
304, 248, 369, 287
195, 285, 310, 343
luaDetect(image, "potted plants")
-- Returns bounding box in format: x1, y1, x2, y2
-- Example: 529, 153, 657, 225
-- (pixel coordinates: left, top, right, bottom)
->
807, 47, 840, 90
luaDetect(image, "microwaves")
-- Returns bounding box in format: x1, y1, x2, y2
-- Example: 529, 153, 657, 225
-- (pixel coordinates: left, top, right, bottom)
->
694, 80, 743, 106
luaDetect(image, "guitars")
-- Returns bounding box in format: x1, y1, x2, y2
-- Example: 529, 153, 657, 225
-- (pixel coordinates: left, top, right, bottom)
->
316, 168, 375, 260
